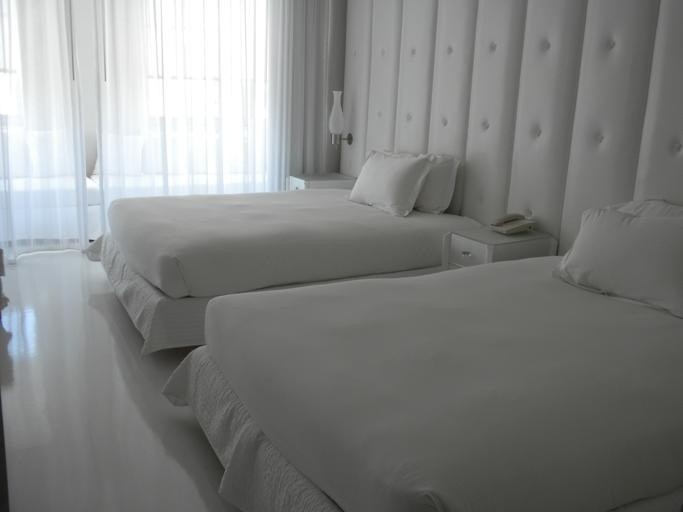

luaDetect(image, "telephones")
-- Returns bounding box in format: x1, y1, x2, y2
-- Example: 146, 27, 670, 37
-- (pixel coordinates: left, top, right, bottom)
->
489, 214, 535, 235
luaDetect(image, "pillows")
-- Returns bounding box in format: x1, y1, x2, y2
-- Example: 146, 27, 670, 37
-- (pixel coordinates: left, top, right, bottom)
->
555, 203, 682, 321
615, 197, 683, 220
378, 149, 463, 216
346, 148, 436, 217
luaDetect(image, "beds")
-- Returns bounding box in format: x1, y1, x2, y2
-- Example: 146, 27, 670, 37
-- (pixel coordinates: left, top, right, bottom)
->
76, 182, 493, 363
155, 250, 683, 511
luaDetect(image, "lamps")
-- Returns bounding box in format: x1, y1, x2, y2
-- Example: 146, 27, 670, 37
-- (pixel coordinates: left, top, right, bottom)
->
325, 90, 354, 147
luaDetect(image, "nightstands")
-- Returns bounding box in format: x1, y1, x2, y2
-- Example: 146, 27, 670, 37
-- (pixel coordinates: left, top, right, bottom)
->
285, 170, 359, 196
443, 222, 558, 273
